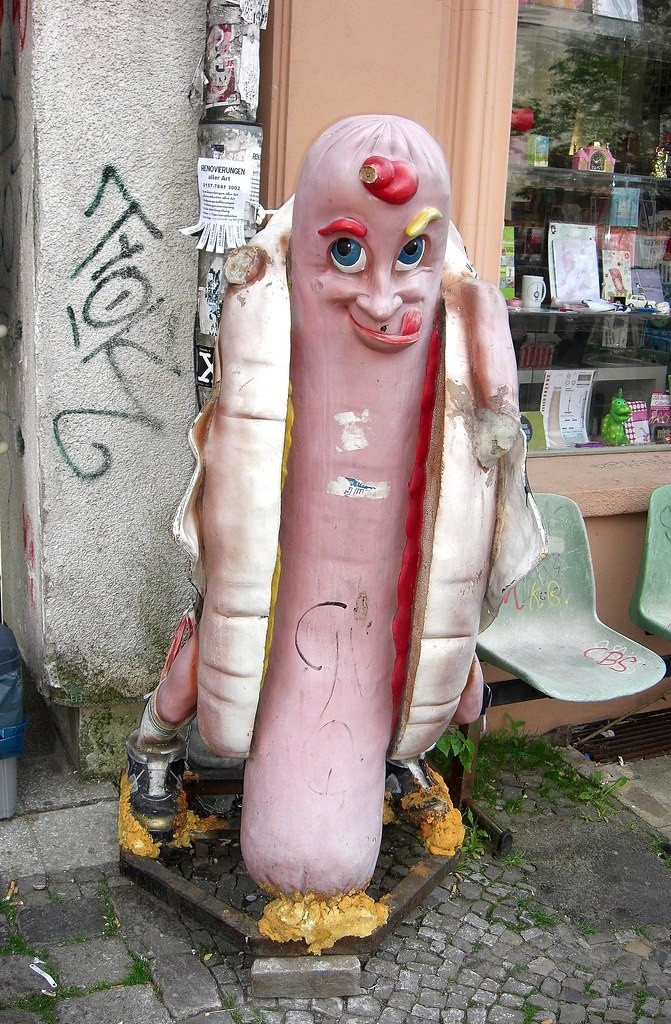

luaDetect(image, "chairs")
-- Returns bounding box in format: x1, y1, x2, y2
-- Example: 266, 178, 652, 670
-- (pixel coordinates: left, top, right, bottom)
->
446, 485, 671, 854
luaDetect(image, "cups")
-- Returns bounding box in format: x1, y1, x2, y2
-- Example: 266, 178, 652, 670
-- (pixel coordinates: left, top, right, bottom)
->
521, 275, 546, 309
512, 107, 534, 132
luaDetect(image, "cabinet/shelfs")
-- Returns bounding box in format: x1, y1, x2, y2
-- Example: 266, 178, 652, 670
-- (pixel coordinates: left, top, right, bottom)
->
497, 164, 670, 318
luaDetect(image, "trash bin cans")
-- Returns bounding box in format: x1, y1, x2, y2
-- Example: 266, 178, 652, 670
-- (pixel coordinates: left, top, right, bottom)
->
0, 624, 29, 820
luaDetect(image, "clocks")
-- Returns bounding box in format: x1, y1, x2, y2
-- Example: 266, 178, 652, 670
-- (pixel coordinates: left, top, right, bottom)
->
572, 141, 617, 174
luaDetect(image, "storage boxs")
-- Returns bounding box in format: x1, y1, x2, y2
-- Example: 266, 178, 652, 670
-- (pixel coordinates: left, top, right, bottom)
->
525, 135, 549, 168
